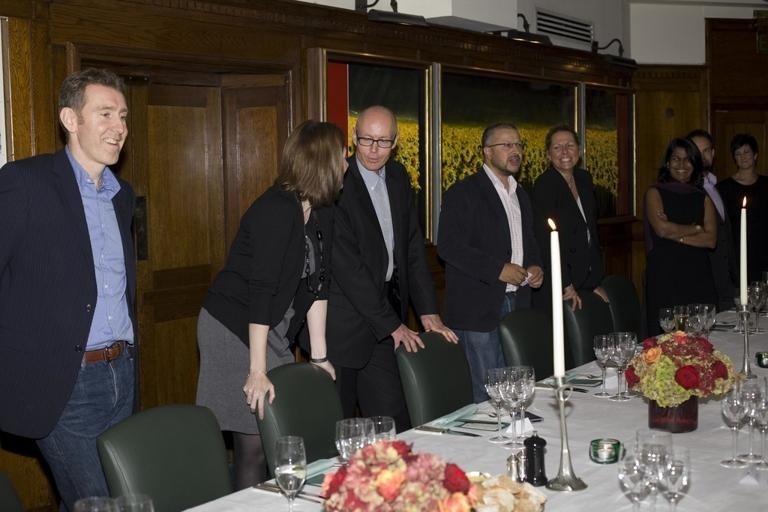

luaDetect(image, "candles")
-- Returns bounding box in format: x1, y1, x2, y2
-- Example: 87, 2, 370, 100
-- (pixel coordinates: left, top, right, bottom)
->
740, 194, 749, 310
545, 216, 568, 379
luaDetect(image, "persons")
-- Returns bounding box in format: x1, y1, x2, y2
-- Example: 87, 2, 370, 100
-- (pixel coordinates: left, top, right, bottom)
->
643, 129, 768, 337
196, 119, 349, 492
436, 121, 544, 403
0, 68, 139, 512
531, 124, 609, 313
295, 105, 460, 433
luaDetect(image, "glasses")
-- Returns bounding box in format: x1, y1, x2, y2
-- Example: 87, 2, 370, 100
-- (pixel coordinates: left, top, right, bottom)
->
355, 129, 398, 149
487, 142, 525, 150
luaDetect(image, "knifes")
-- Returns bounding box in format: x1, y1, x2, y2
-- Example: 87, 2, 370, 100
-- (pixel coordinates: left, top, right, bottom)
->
253, 480, 326, 498
417, 424, 481, 437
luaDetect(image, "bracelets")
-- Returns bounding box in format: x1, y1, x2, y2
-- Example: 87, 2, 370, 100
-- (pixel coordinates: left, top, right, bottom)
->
310, 357, 327, 363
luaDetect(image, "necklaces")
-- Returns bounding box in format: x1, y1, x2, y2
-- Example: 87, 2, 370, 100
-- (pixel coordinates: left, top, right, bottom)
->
301, 217, 326, 300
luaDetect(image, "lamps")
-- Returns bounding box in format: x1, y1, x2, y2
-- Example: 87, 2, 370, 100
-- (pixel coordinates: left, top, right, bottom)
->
493, 8, 554, 47
355, 0, 428, 29
588, 39, 636, 69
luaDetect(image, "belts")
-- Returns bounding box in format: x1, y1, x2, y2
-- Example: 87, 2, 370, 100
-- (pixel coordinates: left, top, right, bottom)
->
82, 340, 128, 363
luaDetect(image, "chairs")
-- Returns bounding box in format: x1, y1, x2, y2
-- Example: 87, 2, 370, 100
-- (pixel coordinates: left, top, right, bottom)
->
252, 356, 348, 477
561, 290, 612, 358
97, 395, 235, 512
597, 271, 642, 348
495, 299, 574, 381
392, 327, 476, 427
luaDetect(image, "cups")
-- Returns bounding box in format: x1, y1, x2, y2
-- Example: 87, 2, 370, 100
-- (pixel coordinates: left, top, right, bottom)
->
336, 414, 394, 466
591, 438, 619, 464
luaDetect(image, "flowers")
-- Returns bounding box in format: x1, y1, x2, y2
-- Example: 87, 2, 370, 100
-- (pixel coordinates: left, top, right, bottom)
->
625, 328, 736, 405
318, 435, 547, 511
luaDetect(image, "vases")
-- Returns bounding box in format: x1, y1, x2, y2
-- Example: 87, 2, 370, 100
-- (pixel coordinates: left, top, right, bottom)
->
644, 397, 700, 432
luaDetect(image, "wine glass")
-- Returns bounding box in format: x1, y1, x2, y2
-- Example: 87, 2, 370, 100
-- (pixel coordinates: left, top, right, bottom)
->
718, 374, 767, 470
271, 437, 308, 511
616, 427, 690, 512
593, 331, 637, 402
485, 364, 536, 450
658, 280, 768, 344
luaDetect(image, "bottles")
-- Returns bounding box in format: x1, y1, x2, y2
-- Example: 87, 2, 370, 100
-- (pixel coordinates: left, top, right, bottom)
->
508, 436, 547, 488
